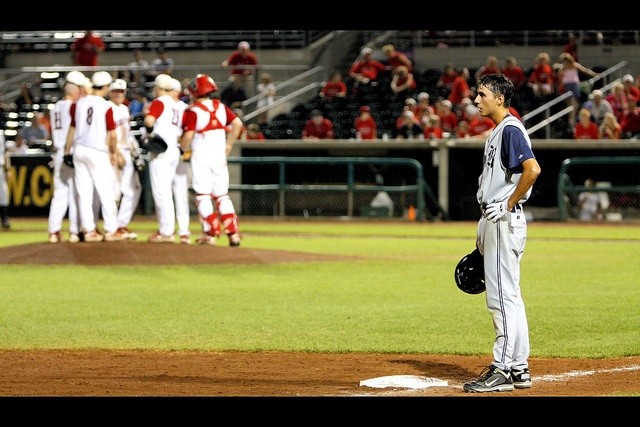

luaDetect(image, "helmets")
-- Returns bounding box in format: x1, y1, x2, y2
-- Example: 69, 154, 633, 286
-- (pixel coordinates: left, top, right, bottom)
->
187, 74, 218, 98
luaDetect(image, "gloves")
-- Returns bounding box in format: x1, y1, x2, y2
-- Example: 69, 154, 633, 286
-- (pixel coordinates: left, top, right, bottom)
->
485, 197, 510, 224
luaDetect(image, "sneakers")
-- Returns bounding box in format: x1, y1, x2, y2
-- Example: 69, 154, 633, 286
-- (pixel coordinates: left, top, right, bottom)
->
84, 231, 104, 243
196, 236, 216, 246
511, 368, 533, 388
147, 232, 175, 244
463, 366, 514, 393
228, 234, 242, 247
68, 234, 80, 245
106, 228, 137, 244
48, 232, 63, 243
180, 234, 192, 247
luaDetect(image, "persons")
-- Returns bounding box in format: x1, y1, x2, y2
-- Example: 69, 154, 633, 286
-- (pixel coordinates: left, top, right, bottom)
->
465, 73, 543, 393
349, 47, 390, 92
396, 92, 465, 140
221, 41, 260, 91
2, 69, 143, 243
573, 110, 599, 140
598, 113, 623, 139
257, 72, 278, 122
150, 46, 174, 76
301, 108, 335, 140
218, 74, 248, 121
112, 50, 150, 81
575, 180, 601, 223
465, 97, 493, 137
169, 76, 190, 244
380, 44, 414, 74
477, 56, 501, 73
503, 55, 525, 88
556, 52, 600, 122
353, 105, 378, 140
527, 52, 556, 96
585, 88, 614, 123
390, 65, 417, 93
437, 60, 470, 91
67, 30, 106, 68
243, 123, 265, 141
613, 74, 640, 131
143, 72, 179, 244
319, 70, 347, 99
179, 73, 244, 247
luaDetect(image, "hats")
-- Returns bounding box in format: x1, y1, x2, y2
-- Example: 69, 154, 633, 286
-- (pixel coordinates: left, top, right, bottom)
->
110, 79, 127, 92
91, 71, 113, 86
65, 71, 85, 87
172, 79, 181, 92
153, 73, 172, 90
81, 77, 91, 90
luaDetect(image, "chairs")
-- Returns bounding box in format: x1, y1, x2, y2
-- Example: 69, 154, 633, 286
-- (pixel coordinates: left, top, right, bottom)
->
1, 68, 172, 143
